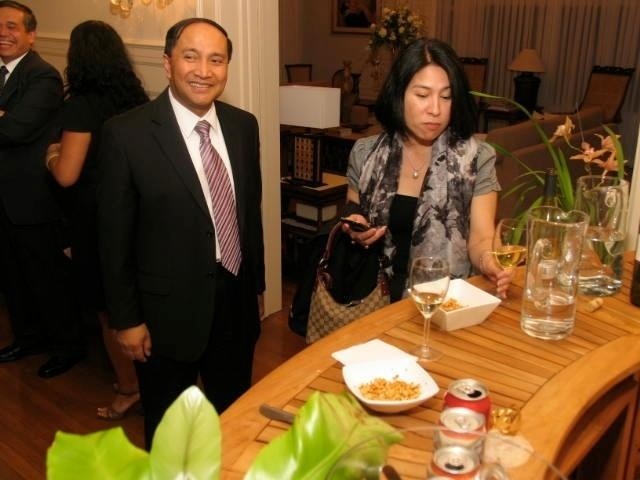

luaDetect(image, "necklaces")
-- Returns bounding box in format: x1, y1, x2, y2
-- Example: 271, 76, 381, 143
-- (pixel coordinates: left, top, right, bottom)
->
399, 146, 437, 182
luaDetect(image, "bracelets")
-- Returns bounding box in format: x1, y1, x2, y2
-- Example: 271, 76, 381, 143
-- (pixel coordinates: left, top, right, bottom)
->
44, 152, 62, 172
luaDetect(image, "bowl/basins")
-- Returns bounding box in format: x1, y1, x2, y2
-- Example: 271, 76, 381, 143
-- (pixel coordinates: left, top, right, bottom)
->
342, 361, 440, 413
407, 278, 502, 332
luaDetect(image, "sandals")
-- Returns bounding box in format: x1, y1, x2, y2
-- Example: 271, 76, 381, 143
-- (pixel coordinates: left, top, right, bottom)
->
96, 388, 142, 421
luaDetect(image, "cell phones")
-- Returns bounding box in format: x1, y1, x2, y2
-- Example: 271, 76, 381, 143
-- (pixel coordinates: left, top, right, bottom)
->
339, 216, 369, 232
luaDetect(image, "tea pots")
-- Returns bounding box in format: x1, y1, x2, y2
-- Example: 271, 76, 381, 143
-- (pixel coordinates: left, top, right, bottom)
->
519, 205, 590, 339
574, 175, 630, 297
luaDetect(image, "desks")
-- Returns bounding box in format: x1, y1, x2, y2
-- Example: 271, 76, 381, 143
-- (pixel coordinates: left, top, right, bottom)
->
288, 98, 387, 176
484, 100, 545, 133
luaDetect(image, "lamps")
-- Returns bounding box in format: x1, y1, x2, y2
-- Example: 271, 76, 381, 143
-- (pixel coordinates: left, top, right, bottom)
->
280, 84, 343, 188
505, 48, 548, 106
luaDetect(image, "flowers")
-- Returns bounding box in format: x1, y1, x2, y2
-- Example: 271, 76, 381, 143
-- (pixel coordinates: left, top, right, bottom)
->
363, 8, 424, 64
471, 88, 632, 281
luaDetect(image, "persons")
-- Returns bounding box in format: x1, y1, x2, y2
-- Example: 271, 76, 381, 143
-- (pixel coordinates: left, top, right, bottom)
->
338, 37, 515, 305
92, 17, 265, 454
43, 18, 152, 425
0, 1, 78, 381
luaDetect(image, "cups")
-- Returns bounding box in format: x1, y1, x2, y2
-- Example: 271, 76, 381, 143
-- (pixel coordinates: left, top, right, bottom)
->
324, 424, 568, 480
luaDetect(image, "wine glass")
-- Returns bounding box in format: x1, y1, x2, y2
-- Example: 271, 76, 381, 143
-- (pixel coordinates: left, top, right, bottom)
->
492, 217, 526, 305
406, 255, 450, 363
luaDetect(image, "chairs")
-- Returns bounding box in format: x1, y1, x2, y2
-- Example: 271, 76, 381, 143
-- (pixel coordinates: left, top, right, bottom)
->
285, 63, 312, 84
453, 56, 487, 138
332, 69, 362, 103
532, 65, 634, 127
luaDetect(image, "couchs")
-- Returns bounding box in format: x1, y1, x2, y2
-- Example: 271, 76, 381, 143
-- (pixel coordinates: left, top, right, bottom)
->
485, 106, 618, 230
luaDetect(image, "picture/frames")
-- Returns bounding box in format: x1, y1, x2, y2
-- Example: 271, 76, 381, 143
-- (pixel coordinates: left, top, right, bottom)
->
330, 0, 381, 34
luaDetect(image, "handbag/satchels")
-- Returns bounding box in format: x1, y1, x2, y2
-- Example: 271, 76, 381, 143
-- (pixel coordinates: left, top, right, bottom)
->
288, 200, 378, 337
305, 222, 391, 344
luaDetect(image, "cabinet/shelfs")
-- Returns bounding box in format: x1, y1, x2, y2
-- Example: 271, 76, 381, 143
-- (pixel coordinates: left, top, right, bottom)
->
281, 172, 349, 276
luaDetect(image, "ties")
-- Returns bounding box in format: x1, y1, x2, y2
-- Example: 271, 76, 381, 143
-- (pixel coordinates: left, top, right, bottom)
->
194, 120, 243, 277
0, 65, 9, 91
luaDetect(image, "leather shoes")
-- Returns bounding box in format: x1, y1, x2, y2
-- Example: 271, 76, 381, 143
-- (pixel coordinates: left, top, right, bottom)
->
38, 358, 70, 378
0, 342, 29, 363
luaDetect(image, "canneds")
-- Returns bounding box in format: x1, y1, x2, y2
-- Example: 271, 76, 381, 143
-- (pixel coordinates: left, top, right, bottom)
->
427, 445, 481, 479
435, 407, 487, 479
443, 377, 491, 431
